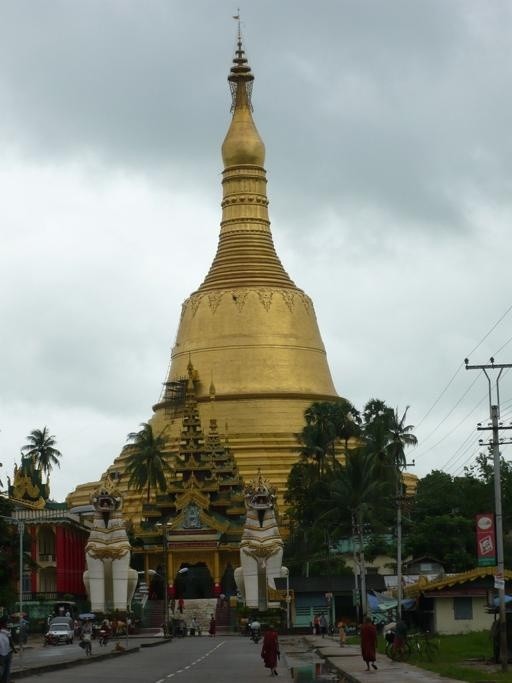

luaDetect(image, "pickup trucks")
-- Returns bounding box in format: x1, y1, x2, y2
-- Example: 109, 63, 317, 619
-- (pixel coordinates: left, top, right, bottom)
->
45, 623, 75, 644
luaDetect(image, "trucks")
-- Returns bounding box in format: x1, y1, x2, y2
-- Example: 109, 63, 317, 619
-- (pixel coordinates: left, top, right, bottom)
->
260, 607, 287, 634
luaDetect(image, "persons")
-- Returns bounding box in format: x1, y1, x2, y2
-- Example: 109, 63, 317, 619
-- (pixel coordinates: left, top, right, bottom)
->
250, 618, 262, 630
209, 617, 217, 637
177, 596, 186, 613
170, 598, 175, 615
314, 613, 321, 635
360, 615, 379, 671
390, 618, 408, 663
73, 617, 142, 655
261, 624, 281, 676
1, 614, 19, 683
319, 611, 329, 638
191, 616, 202, 636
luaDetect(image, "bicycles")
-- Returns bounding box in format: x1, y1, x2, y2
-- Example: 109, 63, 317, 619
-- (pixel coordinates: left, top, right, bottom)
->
83, 632, 93, 656
385, 628, 441, 663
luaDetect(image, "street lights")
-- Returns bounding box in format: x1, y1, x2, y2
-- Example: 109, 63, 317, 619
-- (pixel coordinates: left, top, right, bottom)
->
17, 520, 24, 622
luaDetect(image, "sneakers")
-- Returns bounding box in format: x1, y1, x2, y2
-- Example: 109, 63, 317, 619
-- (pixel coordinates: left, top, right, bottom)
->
274, 670, 278, 675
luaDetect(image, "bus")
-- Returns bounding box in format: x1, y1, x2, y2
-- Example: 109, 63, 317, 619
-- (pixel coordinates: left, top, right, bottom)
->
48, 601, 79, 624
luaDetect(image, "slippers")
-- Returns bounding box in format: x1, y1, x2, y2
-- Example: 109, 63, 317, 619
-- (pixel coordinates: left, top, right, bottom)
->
372, 664, 378, 669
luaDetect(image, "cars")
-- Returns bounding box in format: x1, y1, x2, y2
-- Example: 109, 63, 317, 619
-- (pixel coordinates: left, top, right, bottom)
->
7, 625, 28, 644
168, 619, 187, 636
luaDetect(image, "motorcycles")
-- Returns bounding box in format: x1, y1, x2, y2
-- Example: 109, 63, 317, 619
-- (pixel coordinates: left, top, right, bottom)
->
99, 629, 111, 646
248, 623, 262, 644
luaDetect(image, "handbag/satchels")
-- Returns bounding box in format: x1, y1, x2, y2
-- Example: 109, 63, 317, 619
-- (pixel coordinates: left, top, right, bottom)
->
385, 633, 395, 642
84, 634, 90, 642
